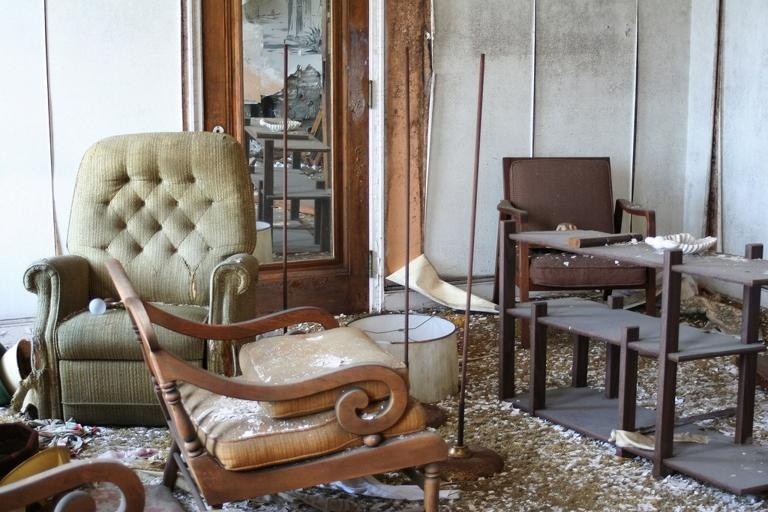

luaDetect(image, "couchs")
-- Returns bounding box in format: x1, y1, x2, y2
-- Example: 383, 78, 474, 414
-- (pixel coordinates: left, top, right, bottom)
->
23, 132, 258, 428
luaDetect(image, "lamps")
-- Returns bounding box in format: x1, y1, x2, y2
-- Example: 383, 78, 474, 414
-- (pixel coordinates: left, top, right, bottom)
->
346, 312, 459, 432
253, 220, 274, 267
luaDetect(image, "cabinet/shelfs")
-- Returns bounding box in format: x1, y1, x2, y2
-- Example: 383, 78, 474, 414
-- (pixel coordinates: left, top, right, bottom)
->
245, 125, 330, 254
499, 221, 768, 495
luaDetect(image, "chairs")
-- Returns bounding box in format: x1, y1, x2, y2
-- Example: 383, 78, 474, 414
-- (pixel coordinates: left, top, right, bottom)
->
0, 462, 187, 512
493, 157, 655, 350
104, 260, 448, 512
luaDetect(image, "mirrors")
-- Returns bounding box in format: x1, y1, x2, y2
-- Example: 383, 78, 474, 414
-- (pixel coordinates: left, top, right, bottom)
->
201, 0, 371, 272
